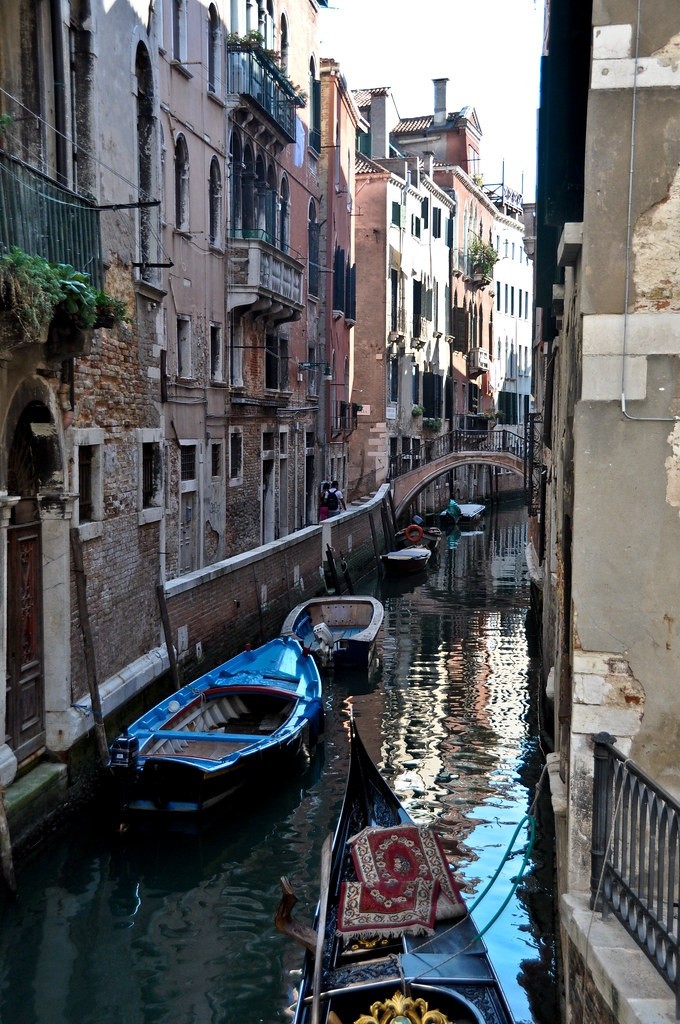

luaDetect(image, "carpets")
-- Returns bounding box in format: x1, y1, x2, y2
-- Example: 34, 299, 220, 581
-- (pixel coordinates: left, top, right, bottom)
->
334, 828, 468, 944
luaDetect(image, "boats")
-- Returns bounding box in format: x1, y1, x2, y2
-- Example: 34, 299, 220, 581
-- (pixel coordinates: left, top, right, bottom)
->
277, 718, 517, 1024
277, 593, 385, 674
103, 633, 323, 815
439, 499, 486, 525
379, 544, 433, 574
393, 515, 442, 553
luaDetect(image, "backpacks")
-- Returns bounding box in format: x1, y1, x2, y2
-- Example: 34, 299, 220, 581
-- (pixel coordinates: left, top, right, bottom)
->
327, 489, 338, 510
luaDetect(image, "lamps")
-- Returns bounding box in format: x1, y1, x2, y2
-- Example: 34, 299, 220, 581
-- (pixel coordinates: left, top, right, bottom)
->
298, 360, 332, 381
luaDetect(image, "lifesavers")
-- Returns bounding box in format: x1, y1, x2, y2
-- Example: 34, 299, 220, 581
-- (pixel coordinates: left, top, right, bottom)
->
406, 525, 423, 542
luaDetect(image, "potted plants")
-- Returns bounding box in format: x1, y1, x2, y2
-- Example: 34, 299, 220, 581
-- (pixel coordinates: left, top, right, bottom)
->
468, 235, 500, 280
226, 32, 241, 49
238, 35, 248, 48
248, 29, 267, 47
412, 406, 423, 419
471, 173, 484, 189
94, 289, 135, 332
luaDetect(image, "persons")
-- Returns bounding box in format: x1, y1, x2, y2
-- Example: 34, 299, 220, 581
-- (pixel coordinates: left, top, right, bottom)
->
317, 480, 346, 521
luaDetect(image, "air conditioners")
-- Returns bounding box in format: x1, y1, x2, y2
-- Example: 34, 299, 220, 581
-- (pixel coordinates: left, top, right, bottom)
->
469, 347, 490, 371
